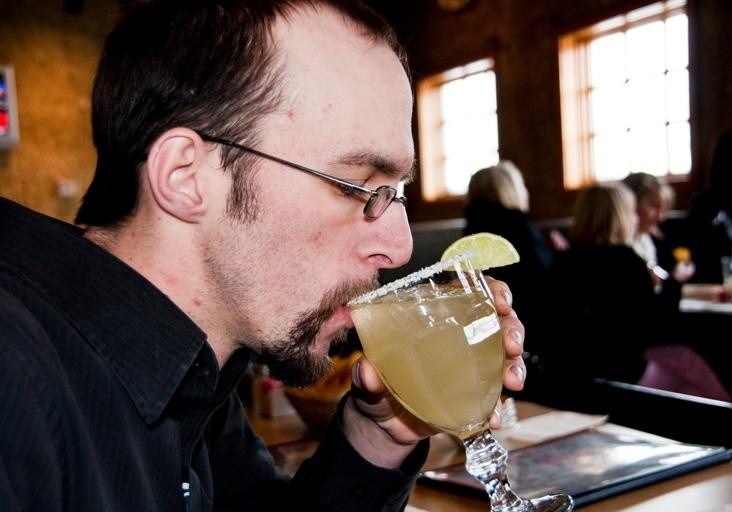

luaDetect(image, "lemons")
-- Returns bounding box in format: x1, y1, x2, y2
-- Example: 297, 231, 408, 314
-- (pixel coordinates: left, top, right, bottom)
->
440, 231, 521, 274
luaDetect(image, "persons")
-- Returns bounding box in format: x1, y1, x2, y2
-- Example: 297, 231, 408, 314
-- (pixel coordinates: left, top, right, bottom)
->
0, 0, 527, 512
462, 160, 732, 404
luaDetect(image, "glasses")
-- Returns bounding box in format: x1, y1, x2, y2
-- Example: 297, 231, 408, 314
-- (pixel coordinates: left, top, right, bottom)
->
187, 123, 413, 222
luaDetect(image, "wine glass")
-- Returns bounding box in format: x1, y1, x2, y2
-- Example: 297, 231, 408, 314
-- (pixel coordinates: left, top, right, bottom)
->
345, 251, 578, 512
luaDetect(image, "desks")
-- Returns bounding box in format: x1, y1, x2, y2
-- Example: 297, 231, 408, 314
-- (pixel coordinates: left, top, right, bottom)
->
277, 397, 732, 512
